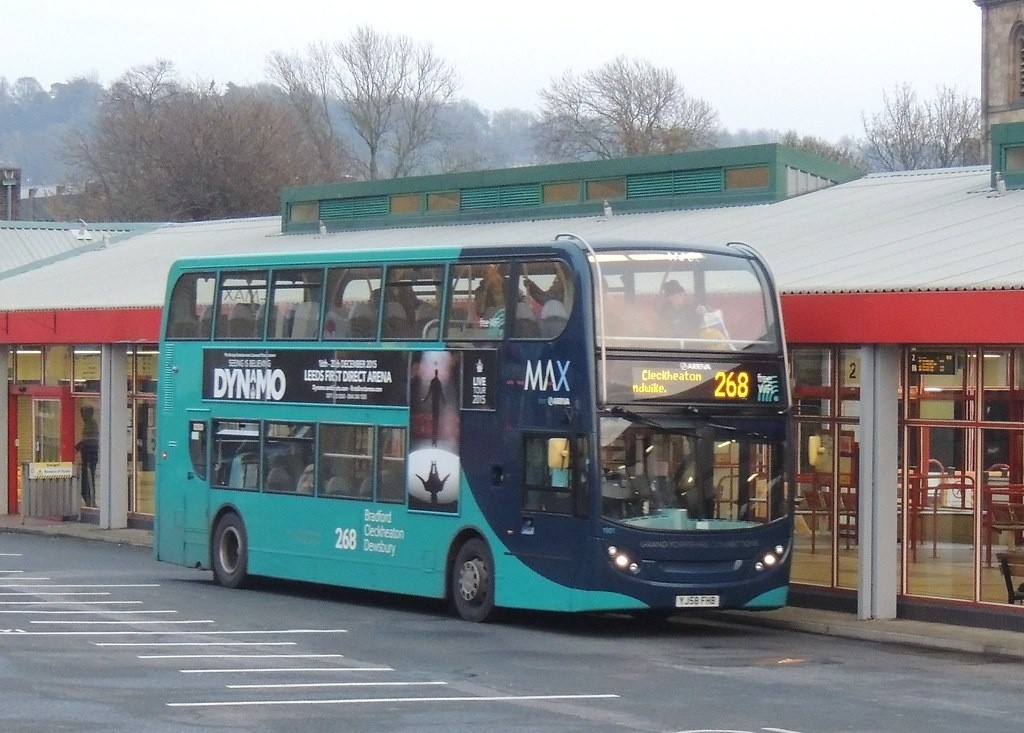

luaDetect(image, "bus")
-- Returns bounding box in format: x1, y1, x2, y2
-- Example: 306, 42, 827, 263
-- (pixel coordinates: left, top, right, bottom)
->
148, 239, 802, 626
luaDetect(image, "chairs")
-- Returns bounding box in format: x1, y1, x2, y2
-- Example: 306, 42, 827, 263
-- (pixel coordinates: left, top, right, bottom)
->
996, 551, 1024, 606
268, 465, 384, 499
175, 297, 569, 341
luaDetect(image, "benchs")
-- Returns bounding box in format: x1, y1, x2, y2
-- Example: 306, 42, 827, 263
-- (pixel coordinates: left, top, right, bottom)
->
991, 502, 1024, 546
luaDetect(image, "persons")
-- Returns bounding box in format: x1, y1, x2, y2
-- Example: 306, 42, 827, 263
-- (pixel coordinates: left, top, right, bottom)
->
370, 266, 428, 322
663, 279, 705, 338
522, 273, 564, 307
75, 405, 98, 507
475, 263, 511, 316
